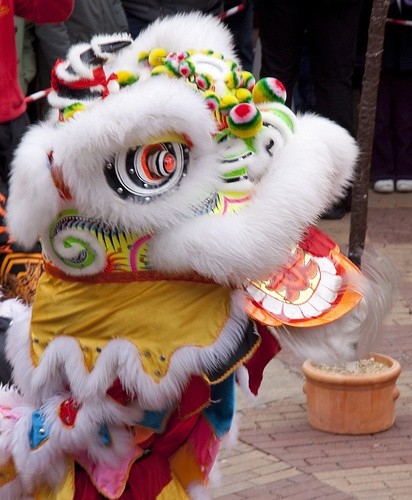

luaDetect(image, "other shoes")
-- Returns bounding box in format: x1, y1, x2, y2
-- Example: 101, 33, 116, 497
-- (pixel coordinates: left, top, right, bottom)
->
397, 179, 412, 193
374, 179, 394, 193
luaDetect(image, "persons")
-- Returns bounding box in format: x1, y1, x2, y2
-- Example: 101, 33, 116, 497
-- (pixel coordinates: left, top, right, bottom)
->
134, 0, 258, 81
371, 0, 412, 194
32, 1, 130, 124
1, 1, 76, 188
251, 3, 372, 220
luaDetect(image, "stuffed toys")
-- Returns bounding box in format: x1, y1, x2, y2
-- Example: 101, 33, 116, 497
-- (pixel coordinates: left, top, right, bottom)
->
1, 11, 398, 499
